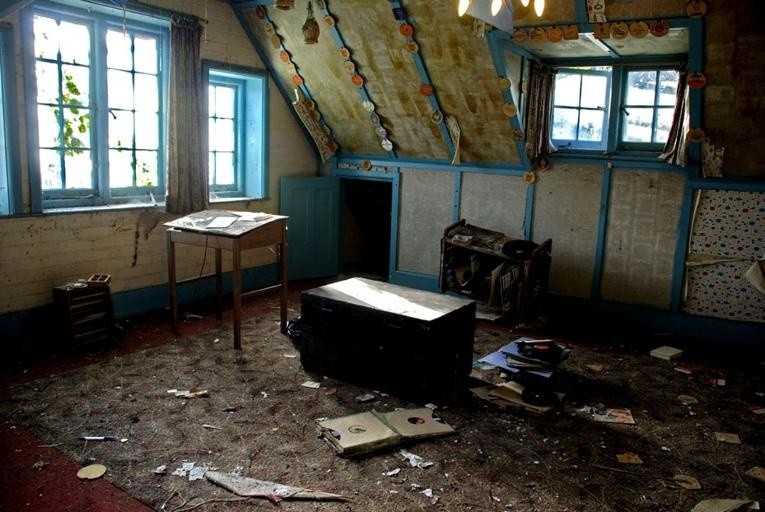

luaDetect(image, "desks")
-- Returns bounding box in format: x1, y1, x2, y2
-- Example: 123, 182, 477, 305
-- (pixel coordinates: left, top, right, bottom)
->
162, 211, 292, 352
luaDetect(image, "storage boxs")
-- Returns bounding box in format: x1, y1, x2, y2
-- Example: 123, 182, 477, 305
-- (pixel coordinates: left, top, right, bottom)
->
300, 278, 477, 397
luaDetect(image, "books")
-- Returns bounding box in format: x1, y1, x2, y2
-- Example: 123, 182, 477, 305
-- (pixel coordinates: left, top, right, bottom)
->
314, 405, 458, 457
649, 344, 685, 362
482, 260, 522, 315
469, 332, 574, 417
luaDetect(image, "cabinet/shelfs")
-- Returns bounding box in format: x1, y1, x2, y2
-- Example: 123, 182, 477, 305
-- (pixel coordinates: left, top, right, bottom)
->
52, 281, 117, 351
436, 217, 555, 311
